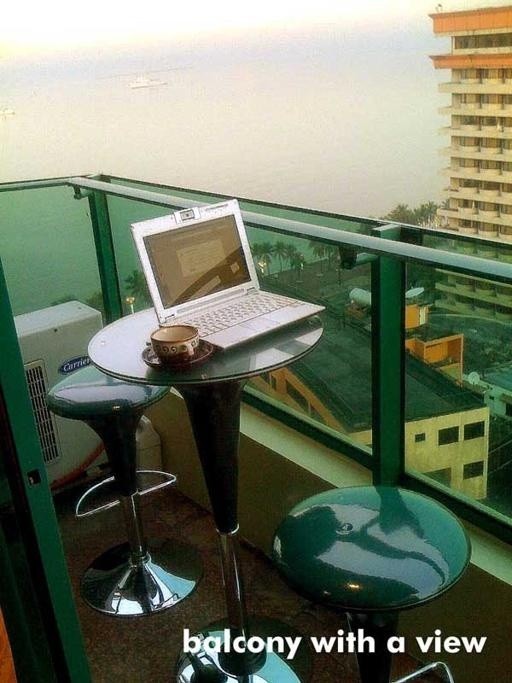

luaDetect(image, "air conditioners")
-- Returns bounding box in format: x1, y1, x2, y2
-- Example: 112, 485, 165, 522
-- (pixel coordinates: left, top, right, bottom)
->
9, 299, 128, 501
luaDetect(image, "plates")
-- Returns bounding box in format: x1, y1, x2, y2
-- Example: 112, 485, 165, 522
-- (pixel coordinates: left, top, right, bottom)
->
142, 340, 215, 372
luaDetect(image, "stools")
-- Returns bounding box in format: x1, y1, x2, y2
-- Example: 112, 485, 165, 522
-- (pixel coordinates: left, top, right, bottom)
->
270, 483, 473, 682
42, 364, 207, 621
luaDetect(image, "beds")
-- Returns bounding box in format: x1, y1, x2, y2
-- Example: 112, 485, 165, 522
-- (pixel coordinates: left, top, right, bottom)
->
86, 290, 325, 682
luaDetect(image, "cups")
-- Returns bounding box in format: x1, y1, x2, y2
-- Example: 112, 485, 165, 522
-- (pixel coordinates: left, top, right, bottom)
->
150, 324, 199, 363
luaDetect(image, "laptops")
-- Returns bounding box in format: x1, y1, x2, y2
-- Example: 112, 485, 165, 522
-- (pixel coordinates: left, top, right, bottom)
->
130, 198, 326, 354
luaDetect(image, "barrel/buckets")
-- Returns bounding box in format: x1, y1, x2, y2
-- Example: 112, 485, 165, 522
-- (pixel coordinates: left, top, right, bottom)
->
134, 416, 162, 493
